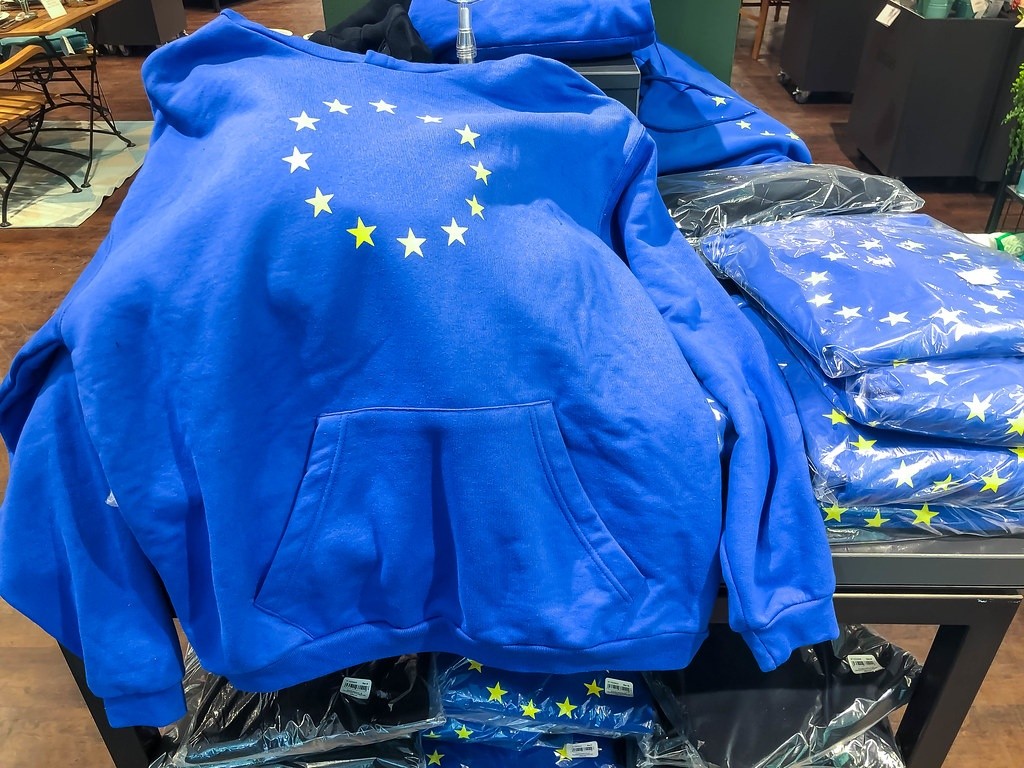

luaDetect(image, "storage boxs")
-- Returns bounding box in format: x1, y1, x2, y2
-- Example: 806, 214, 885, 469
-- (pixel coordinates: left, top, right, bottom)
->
570, 56, 642, 116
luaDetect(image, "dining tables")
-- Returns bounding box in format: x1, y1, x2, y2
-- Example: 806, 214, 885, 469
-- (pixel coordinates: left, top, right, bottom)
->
0, 0, 136, 188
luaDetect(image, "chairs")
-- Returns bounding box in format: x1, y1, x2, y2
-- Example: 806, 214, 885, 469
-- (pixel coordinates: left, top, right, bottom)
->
0, 46, 82, 228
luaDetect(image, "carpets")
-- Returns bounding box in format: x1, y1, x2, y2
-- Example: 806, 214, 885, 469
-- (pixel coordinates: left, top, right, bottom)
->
0, 120, 154, 228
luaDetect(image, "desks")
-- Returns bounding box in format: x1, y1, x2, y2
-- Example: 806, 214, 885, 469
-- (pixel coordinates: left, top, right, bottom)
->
58, 537, 1024, 768
